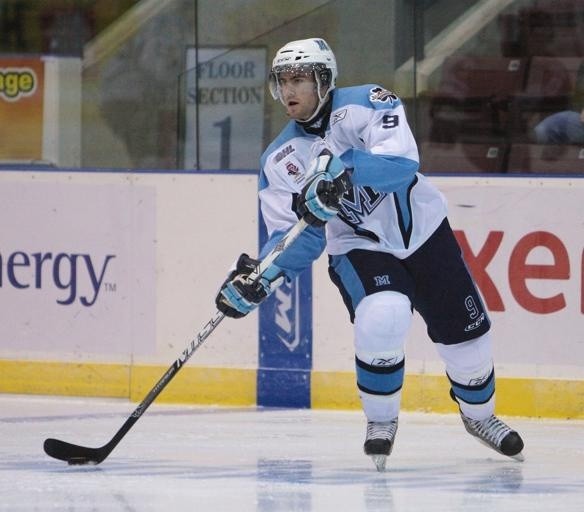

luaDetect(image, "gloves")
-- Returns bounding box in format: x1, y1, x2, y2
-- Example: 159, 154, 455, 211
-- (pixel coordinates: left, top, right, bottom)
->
295, 148, 351, 227
215, 253, 285, 319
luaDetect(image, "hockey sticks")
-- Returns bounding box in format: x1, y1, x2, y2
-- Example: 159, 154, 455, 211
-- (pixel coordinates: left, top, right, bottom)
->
43, 217, 309, 465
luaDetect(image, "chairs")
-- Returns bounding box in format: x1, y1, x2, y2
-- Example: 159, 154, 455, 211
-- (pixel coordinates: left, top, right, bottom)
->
421, 0, 584, 174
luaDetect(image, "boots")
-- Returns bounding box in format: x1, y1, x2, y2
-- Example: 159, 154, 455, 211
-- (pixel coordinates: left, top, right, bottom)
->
364, 416, 397, 455
451, 387, 524, 456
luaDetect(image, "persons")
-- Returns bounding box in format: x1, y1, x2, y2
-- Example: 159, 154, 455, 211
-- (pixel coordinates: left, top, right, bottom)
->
455, 11, 583, 174
216, 36, 524, 458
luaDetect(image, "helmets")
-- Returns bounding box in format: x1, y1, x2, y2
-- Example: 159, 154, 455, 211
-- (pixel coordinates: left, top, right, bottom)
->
268, 38, 338, 90
